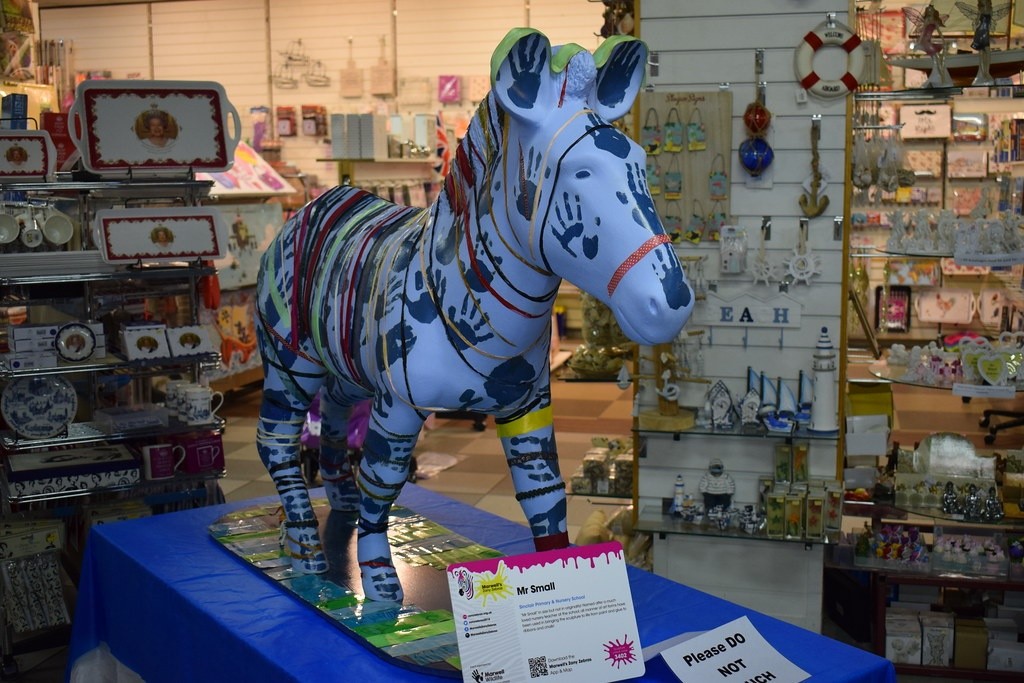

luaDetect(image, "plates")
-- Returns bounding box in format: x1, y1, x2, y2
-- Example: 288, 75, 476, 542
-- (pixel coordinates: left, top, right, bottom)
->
1, 374, 78, 438
54, 321, 97, 364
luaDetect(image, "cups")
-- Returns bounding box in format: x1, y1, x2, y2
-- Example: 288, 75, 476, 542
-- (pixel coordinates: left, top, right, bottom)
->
186, 389, 224, 425
0, 214, 20, 243
15, 204, 43, 249
167, 380, 188, 417
177, 384, 203, 421
35, 209, 74, 246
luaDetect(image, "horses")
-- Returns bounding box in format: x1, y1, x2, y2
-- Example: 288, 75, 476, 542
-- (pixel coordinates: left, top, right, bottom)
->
254, 25, 695, 606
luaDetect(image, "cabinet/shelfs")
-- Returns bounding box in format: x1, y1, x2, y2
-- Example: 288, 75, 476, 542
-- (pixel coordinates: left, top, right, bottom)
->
558, 360, 635, 506
1, 180, 225, 683
631, 363, 1023, 682
206, 153, 446, 408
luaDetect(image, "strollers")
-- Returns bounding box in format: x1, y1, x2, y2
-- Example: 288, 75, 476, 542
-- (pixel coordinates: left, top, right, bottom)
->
303, 376, 416, 489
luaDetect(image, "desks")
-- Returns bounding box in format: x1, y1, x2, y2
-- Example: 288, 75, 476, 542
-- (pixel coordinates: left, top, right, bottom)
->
68, 479, 895, 683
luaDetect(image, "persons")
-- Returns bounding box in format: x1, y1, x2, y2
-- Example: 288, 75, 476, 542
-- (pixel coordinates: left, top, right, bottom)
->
140, 339, 156, 352
183, 335, 197, 348
964, 484, 982, 517
139, 111, 175, 146
700, 458, 734, 509
154, 230, 171, 247
68, 336, 81, 351
943, 481, 961, 514
985, 487, 1003, 519
9, 147, 26, 166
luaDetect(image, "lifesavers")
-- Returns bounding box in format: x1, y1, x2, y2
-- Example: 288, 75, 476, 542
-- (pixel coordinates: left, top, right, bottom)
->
797, 28, 865, 98
